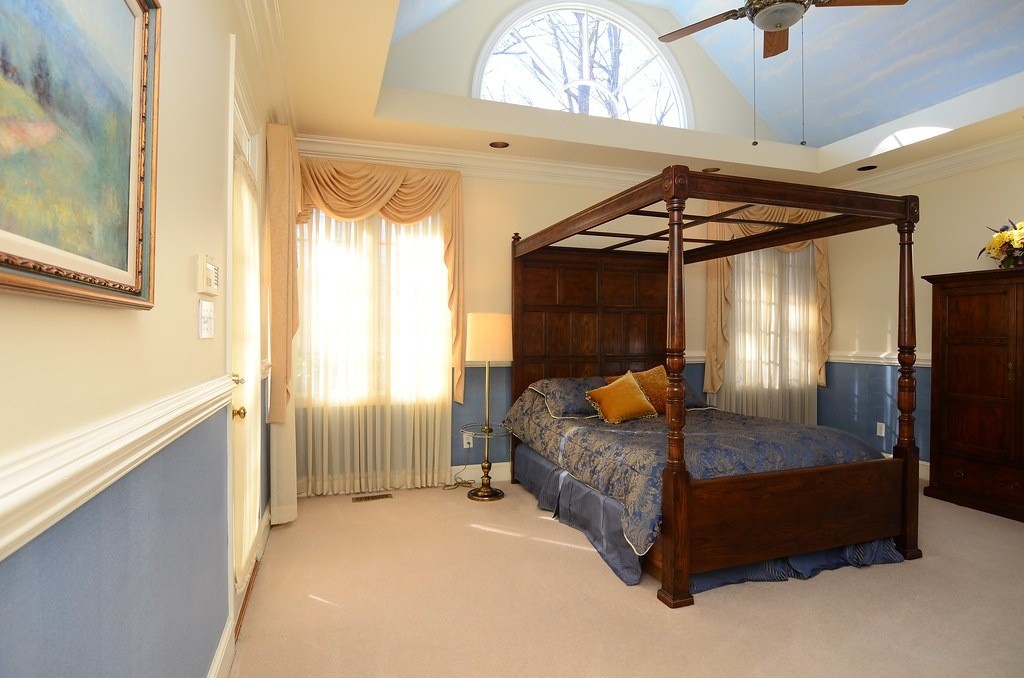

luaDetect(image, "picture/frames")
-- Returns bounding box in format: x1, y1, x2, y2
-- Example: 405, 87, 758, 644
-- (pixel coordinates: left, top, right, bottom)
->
0, 0, 164, 310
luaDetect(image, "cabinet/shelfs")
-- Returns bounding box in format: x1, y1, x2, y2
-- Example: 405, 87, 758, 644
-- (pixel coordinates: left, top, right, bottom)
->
920, 268, 1024, 523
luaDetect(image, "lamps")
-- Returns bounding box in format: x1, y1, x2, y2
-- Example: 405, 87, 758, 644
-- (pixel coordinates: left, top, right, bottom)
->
460, 312, 514, 502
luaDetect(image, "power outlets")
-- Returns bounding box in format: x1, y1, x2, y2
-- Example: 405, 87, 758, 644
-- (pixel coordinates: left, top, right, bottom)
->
200, 299, 216, 341
462, 433, 474, 449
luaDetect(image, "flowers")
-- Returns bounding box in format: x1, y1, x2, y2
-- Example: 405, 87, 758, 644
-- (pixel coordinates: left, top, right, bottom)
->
976, 217, 1024, 269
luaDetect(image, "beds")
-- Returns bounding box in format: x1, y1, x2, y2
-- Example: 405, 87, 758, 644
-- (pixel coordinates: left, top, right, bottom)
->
498, 165, 922, 609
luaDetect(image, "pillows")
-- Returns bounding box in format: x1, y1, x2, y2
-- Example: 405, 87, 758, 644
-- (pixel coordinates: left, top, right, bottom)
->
530, 377, 608, 419
585, 370, 659, 425
603, 364, 670, 415
685, 378, 718, 411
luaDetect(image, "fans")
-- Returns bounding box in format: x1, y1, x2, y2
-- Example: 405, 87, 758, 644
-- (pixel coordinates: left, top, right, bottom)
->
657, 0, 907, 61
